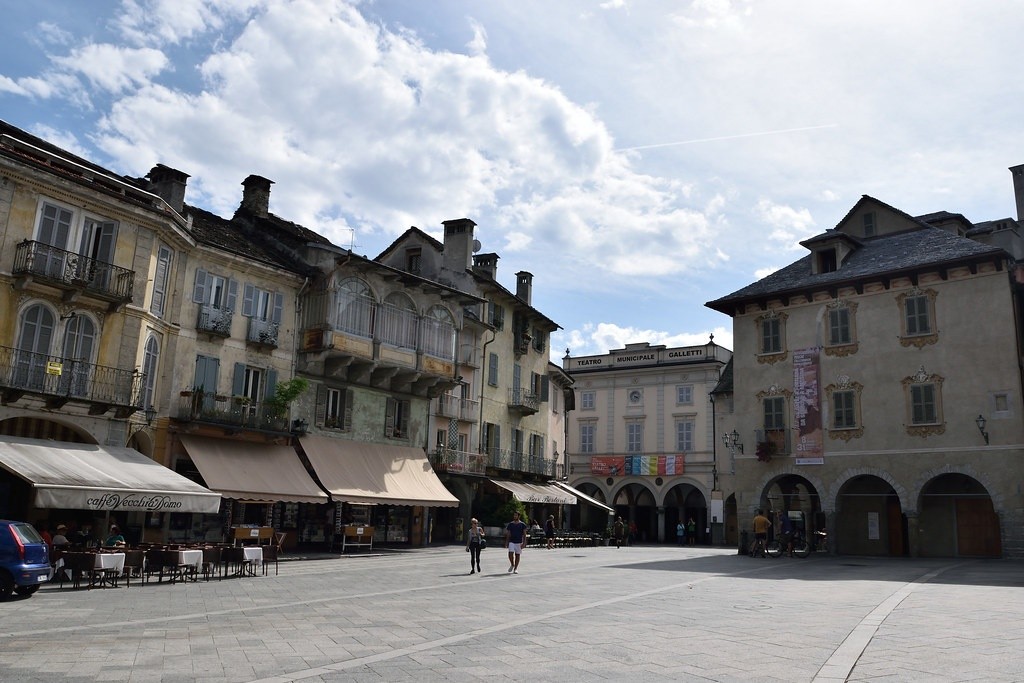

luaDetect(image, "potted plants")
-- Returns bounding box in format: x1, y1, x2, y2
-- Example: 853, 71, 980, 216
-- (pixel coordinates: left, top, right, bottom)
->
621, 524, 629, 545
603, 527, 612, 546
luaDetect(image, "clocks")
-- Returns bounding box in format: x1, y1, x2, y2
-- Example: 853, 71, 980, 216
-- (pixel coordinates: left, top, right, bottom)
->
630, 391, 641, 402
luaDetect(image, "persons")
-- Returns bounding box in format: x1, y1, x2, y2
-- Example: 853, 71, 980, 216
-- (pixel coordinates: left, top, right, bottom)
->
752, 511, 772, 558
615, 516, 637, 549
50, 525, 73, 549
105, 527, 126, 546
532, 519, 539, 529
677, 520, 685, 546
505, 512, 528, 573
687, 516, 696, 548
776, 509, 794, 558
546, 514, 557, 550
465, 518, 487, 575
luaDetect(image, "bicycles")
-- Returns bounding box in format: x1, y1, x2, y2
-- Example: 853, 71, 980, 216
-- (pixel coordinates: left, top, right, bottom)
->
766, 532, 811, 559
750, 540, 762, 558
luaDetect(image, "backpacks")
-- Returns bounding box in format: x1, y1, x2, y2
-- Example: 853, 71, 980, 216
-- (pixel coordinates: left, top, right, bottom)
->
547, 520, 553, 529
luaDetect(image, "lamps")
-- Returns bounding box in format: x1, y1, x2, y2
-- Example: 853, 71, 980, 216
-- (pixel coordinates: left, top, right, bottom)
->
712, 466, 719, 482
553, 451, 560, 462
432, 441, 444, 453
731, 429, 743, 454
976, 414, 990, 445
128, 405, 157, 437
722, 433, 734, 447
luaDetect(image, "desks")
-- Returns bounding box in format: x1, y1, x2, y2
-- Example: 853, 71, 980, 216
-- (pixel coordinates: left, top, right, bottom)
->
221, 546, 263, 576
143, 549, 203, 584
60, 552, 126, 590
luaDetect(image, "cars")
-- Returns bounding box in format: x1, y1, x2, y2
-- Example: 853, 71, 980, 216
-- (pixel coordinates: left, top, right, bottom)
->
0, 519, 52, 600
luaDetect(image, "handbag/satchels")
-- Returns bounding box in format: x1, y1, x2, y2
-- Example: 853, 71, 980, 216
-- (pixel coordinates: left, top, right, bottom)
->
479, 539, 487, 549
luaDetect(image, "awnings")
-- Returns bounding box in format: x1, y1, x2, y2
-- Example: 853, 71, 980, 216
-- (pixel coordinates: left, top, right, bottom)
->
0, 434, 461, 514
489, 480, 616, 515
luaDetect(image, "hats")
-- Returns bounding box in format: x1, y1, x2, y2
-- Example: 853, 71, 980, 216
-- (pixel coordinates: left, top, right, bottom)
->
110, 524, 118, 531
57, 525, 68, 530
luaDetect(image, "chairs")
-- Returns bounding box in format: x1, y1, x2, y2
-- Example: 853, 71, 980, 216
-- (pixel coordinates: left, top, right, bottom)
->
530, 529, 593, 548
51, 541, 279, 591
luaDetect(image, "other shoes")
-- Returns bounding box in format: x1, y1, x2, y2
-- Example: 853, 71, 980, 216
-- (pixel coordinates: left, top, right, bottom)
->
513, 571, 518, 574
470, 570, 475, 574
508, 565, 515, 572
477, 566, 480, 572
546, 545, 554, 549
